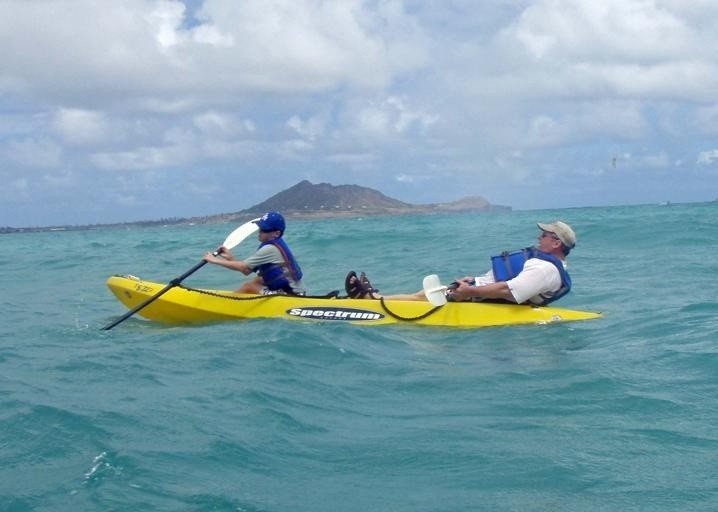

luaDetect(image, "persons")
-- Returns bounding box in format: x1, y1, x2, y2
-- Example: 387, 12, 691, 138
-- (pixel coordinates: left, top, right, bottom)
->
202, 211, 307, 299
344, 219, 576, 307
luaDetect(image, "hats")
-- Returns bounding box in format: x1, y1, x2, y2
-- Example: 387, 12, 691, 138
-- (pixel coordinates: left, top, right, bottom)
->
251, 212, 285, 231
538, 221, 576, 248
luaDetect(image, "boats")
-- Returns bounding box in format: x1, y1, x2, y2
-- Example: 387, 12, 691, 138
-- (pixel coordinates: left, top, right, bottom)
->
106, 272, 608, 329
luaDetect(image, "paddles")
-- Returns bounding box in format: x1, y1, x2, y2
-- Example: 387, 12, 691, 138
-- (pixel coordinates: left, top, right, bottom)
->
103, 219, 261, 331
421, 274, 477, 307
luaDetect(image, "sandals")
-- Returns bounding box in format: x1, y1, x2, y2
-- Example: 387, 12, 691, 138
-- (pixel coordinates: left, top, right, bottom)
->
345, 271, 367, 299
360, 272, 372, 293
